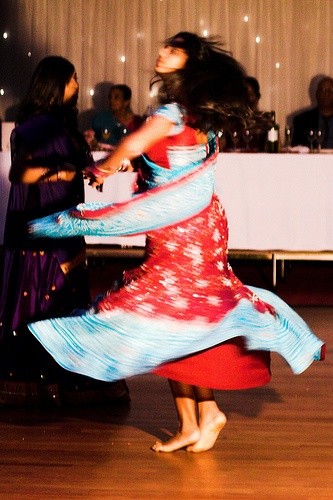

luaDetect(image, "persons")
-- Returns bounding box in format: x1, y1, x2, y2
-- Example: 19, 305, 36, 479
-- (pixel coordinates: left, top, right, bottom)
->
227, 77, 280, 151
27, 31, 328, 453
290, 77, 332, 151
0, 56, 133, 412
83, 84, 144, 149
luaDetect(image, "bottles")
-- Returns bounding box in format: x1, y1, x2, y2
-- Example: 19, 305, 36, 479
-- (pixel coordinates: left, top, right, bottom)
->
144, 106, 153, 121
267, 111, 279, 153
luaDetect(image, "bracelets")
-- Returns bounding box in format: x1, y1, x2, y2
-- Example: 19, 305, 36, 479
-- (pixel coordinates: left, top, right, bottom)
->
95, 159, 118, 174
47, 168, 61, 182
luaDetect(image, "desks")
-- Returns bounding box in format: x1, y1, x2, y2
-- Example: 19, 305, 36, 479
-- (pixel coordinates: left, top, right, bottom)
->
0, 150, 333, 286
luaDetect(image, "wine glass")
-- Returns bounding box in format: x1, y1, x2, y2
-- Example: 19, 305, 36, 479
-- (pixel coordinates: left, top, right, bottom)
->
232, 128, 240, 152
307, 129, 316, 153
243, 129, 253, 151
314, 129, 324, 153
